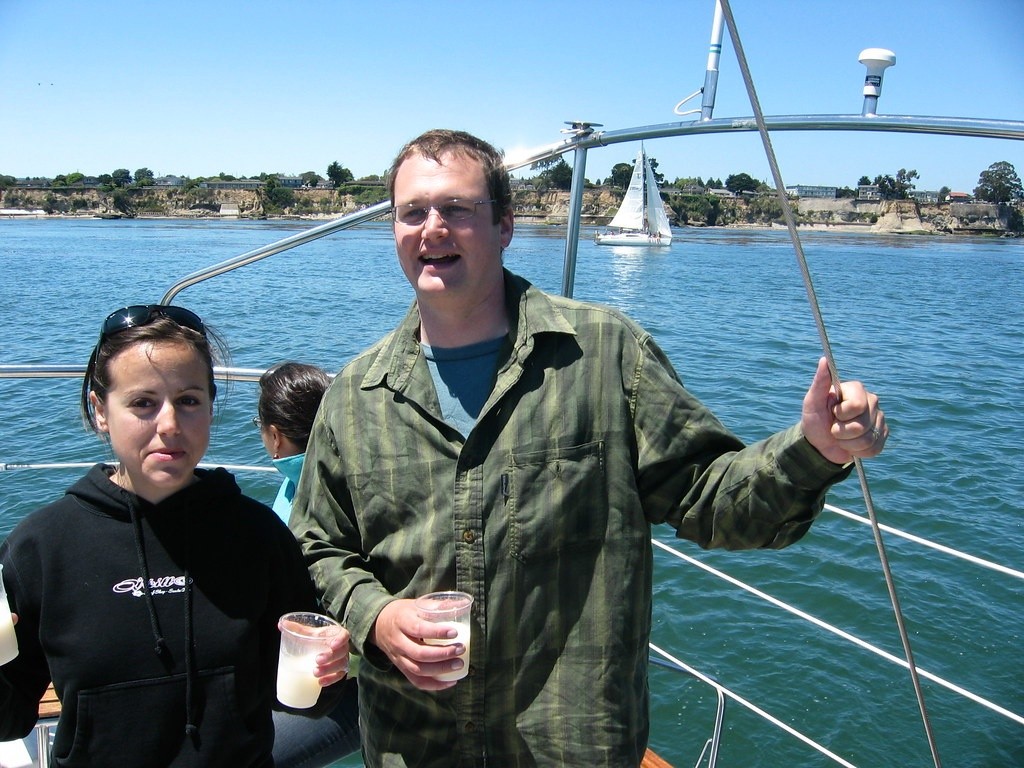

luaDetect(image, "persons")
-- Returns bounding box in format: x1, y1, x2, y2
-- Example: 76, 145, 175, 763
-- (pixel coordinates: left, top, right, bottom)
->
0, 303, 352, 768
258, 362, 363, 768
288, 130, 890, 768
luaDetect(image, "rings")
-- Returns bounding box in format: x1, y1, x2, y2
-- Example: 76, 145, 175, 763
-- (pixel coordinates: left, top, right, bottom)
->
871, 426, 880, 440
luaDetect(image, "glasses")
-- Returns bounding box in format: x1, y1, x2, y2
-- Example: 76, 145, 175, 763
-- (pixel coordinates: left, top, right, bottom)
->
253, 414, 263, 428
391, 199, 497, 225
94, 304, 208, 368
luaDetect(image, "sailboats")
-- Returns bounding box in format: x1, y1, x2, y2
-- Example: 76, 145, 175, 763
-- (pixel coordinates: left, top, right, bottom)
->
593, 139, 674, 248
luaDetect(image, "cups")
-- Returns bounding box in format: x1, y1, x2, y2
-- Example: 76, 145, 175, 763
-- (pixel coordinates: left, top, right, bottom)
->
413, 590, 474, 682
277, 611, 342, 709
1, 564, 19, 666
